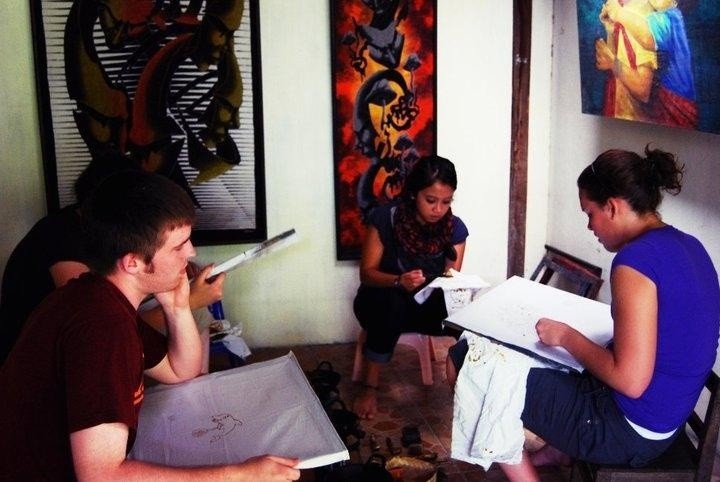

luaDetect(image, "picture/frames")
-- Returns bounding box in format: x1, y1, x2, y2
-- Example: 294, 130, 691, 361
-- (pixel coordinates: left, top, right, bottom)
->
27, 1, 269, 247
327, 0, 440, 263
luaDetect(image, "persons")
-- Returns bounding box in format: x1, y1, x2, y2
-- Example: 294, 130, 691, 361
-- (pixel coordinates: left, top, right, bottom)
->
1, 148, 226, 330
602, 0, 699, 130
593, 1, 659, 122
445, 140, 720, 482
352, 153, 468, 421
0, 173, 303, 481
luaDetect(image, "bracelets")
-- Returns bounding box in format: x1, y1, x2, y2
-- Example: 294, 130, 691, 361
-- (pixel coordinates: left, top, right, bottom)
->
612, 58, 621, 78
394, 274, 401, 289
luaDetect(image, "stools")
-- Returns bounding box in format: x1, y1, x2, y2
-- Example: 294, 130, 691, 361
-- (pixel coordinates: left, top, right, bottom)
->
352, 327, 437, 386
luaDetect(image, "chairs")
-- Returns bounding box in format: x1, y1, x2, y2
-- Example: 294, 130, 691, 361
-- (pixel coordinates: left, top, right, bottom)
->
574, 369, 720, 482
